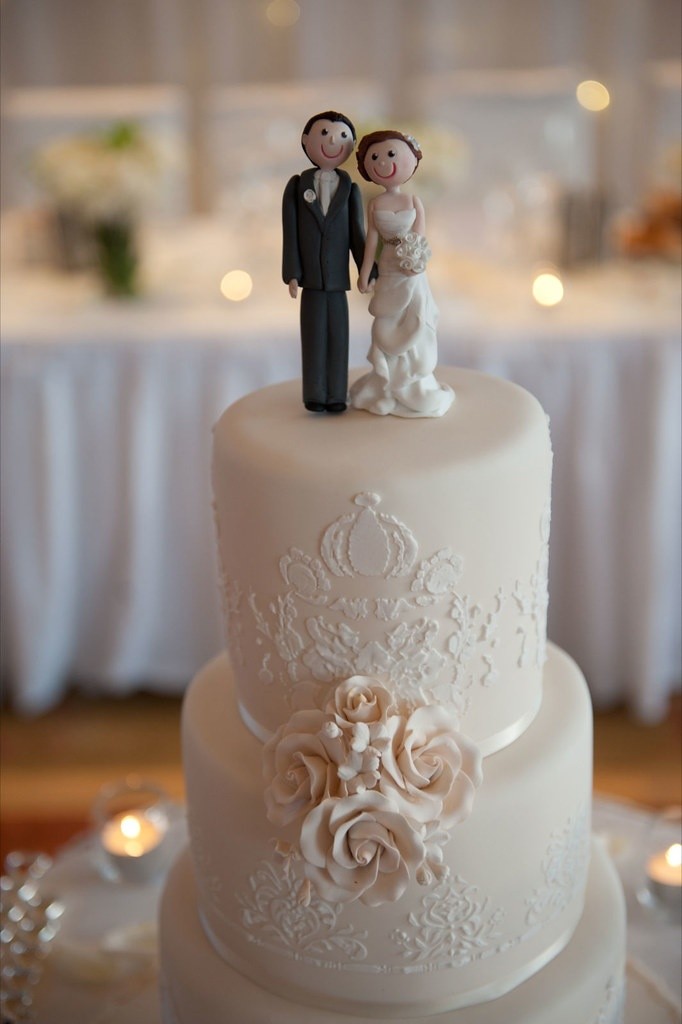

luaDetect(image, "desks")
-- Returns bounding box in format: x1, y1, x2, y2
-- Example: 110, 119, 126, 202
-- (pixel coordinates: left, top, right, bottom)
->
1, 277, 680, 719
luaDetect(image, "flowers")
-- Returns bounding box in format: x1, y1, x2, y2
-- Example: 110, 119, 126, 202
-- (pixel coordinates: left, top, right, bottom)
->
29, 127, 165, 298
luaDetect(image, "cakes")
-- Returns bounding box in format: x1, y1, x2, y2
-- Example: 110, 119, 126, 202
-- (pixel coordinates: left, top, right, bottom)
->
157, 112, 628, 1024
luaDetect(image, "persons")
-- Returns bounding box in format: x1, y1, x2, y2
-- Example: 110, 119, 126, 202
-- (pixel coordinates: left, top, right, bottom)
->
281, 110, 456, 418
